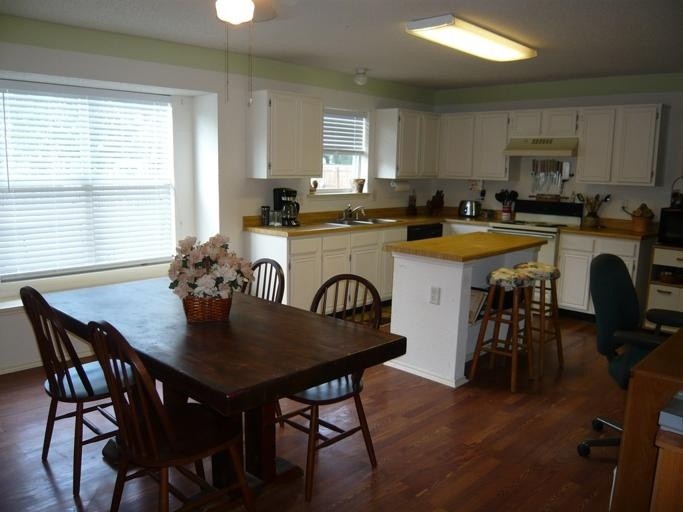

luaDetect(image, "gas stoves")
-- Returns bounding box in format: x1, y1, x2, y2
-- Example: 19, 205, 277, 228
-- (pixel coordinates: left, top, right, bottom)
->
489, 211, 582, 234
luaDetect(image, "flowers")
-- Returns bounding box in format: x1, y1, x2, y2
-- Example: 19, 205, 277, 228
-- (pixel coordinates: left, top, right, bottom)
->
167, 236, 258, 321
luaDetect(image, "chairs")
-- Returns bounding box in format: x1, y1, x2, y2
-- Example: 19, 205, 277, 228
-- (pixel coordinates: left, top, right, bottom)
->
20, 286, 137, 495
576, 251, 668, 461
241, 257, 286, 428
284, 273, 385, 500
87, 320, 254, 512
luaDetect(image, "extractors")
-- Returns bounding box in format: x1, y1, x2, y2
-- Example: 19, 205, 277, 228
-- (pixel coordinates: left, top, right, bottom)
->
503, 137, 579, 158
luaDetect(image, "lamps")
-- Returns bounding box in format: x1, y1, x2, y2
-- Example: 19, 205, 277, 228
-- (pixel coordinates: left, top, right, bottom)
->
354, 68, 368, 86
353, 98, 369, 126
404, 15, 540, 64
214, 0, 257, 25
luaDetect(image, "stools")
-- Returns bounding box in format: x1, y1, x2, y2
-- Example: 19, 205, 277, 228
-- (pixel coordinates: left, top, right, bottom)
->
467, 268, 537, 391
503, 260, 565, 377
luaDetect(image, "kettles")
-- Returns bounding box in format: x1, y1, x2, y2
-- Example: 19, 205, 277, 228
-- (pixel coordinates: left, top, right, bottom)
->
281, 201, 300, 220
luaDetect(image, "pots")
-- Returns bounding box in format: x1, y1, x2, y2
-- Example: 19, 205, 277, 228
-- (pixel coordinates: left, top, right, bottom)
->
457, 199, 482, 219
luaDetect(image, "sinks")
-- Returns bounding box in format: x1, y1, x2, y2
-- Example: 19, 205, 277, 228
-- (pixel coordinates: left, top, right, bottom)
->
326, 217, 403, 227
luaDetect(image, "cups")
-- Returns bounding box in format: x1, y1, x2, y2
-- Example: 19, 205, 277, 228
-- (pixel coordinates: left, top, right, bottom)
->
261, 205, 270, 225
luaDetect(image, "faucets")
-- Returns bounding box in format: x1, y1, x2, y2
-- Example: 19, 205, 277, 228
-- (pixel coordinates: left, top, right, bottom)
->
343, 203, 365, 221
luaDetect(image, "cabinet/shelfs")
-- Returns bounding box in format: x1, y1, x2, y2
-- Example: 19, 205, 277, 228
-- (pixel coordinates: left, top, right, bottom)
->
508, 105, 580, 156
639, 241, 683, 333
247, 90, 324, 181
442, 110, 506, 183
580, 101, 669, 186
247, 227, 409, 315
375, 106, 439, 183
558, 232, 639, 317
